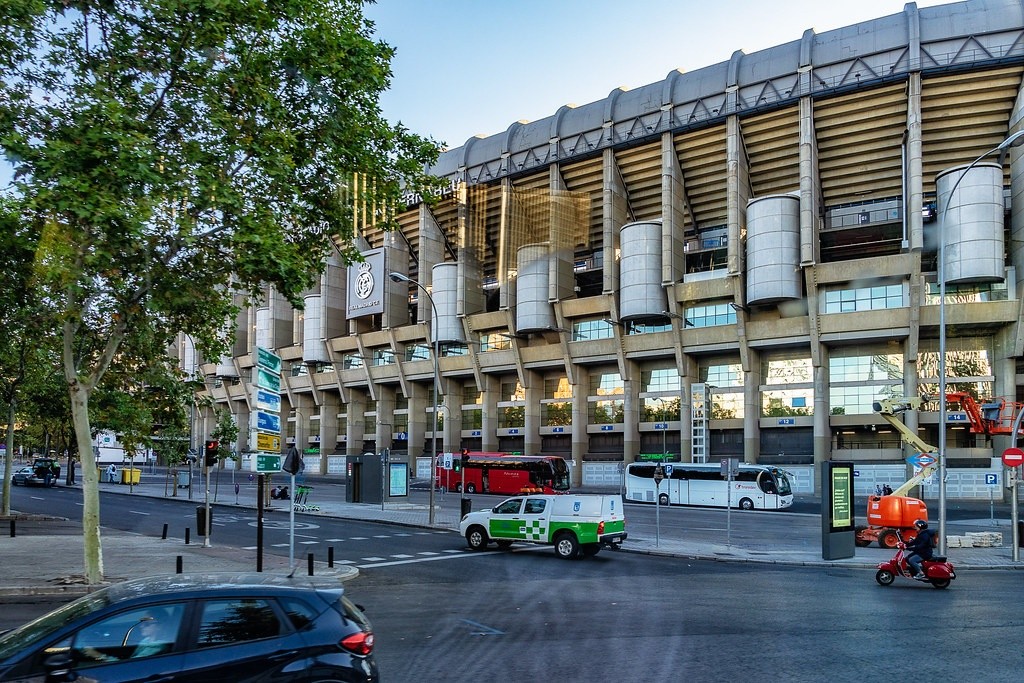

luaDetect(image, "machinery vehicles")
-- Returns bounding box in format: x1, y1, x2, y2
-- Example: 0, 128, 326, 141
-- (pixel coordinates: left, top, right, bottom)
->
855, 392, 1024, 549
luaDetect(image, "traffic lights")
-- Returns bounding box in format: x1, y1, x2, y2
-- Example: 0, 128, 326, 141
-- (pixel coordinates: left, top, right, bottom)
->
206, 440, 218, 466
462, 447, 469, 467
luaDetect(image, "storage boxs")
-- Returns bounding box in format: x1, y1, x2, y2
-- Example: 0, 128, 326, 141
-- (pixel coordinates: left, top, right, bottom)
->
946, 531, 1003, 548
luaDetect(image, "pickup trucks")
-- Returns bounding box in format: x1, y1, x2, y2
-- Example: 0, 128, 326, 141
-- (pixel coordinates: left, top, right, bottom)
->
460, 494, 628, 559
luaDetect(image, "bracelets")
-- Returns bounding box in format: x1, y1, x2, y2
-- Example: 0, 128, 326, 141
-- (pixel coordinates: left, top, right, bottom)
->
96, 654, 104, 660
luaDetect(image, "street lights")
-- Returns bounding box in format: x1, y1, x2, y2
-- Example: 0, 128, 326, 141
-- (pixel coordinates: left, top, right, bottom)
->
290, 410, 303, 459
390, 273, 438, 524
938, 130, 1024, 563
437, 404, 451, 452
652, 397, 665, 461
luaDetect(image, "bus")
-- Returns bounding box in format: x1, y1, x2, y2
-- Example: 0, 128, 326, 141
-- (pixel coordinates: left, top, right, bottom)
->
436, 451, 570, 496
625, 462, 794, 511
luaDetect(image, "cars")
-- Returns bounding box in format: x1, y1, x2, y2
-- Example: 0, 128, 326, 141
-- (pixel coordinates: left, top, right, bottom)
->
180, 449, 197, 463
11, 458, 56, 487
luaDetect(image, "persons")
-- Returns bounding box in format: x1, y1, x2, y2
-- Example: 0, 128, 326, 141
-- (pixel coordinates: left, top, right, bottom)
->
37, 461, 61, 487
273, 486, 290, 500
71, 461, 77, 484
509, 503, 521, 512
106, 462, 117, 485
903, 520, 933, 578
82, 608, 171, 662
874, 484, 893, 496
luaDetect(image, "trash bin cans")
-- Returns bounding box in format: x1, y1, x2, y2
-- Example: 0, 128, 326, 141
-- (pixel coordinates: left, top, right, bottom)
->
97, 466, 123, 484
122, 468, 142, 484
196, 505, 212, 536
178, 472, 190, 488
1018, 520, 1024, 548
461, 498, 471, 520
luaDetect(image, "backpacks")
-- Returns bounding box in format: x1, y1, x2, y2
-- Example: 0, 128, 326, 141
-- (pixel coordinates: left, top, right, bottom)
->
925, 529, 939, 548
111, 467, 116, 472
271, 489, 277, 497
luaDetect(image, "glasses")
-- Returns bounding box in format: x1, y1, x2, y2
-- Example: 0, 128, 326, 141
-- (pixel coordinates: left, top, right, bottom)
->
141, 622, 160, 627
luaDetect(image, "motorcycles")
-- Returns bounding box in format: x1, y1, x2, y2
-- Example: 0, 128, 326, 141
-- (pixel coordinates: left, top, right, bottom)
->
875, 529, 956, 588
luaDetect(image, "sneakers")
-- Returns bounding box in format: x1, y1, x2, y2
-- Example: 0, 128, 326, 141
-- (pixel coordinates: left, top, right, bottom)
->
913, 573, 926, 579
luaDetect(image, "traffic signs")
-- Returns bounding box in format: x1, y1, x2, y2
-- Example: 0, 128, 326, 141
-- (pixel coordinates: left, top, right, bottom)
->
250, 346, 282, 473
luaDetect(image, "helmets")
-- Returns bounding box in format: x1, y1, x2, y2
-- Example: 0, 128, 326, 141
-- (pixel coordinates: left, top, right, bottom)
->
915, 519, 928, 529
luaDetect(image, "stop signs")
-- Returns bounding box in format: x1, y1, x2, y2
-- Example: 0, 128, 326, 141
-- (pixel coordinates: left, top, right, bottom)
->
1002, 447, 1024, 467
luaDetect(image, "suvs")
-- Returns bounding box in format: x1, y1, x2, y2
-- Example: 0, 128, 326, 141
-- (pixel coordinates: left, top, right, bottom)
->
0, 573, 380, 683
